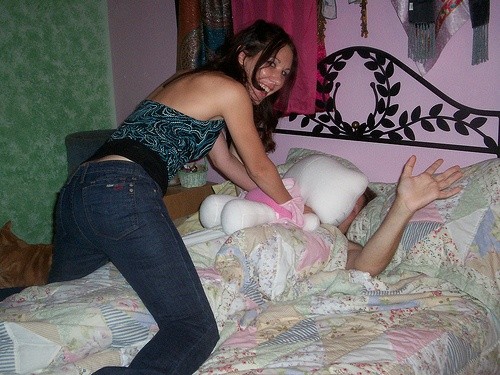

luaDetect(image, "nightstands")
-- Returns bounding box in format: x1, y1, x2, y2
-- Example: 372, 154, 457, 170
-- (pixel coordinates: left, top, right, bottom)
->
161, 177, 219, 220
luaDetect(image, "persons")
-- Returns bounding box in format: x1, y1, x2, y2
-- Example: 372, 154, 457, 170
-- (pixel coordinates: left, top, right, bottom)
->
0, 17, 323, 374
310, 151, 464, 278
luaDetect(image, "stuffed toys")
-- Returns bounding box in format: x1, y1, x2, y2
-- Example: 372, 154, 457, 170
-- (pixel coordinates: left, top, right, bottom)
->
198, 153, 370, 232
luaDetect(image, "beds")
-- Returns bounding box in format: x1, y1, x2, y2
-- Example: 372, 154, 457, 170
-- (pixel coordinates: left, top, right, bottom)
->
1, 193, 499, 375
